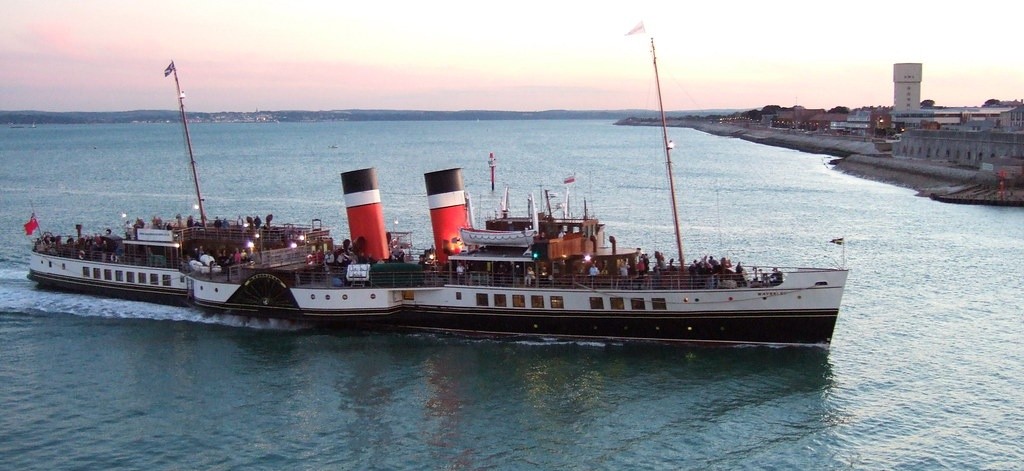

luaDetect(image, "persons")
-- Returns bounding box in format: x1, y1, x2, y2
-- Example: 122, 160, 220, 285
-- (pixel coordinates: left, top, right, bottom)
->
34, 215, 781, 289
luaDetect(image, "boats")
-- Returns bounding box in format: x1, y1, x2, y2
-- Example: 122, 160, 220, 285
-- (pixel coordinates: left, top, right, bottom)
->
26, 36, 849, 351
458, 227, 534, 245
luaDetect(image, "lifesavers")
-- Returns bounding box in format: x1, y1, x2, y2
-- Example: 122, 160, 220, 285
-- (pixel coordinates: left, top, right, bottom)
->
79, 250, 86, 259
306, 254, 314, 265
111, 253, 118, 262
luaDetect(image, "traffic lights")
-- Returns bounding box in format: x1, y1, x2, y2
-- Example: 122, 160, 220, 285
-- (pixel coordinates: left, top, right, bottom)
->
532, 244, 540, 261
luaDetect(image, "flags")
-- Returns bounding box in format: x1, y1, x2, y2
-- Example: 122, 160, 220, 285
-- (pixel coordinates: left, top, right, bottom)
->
165, 63, 174, 77
625, 20, 645, 37
24, 217, 38, 235
